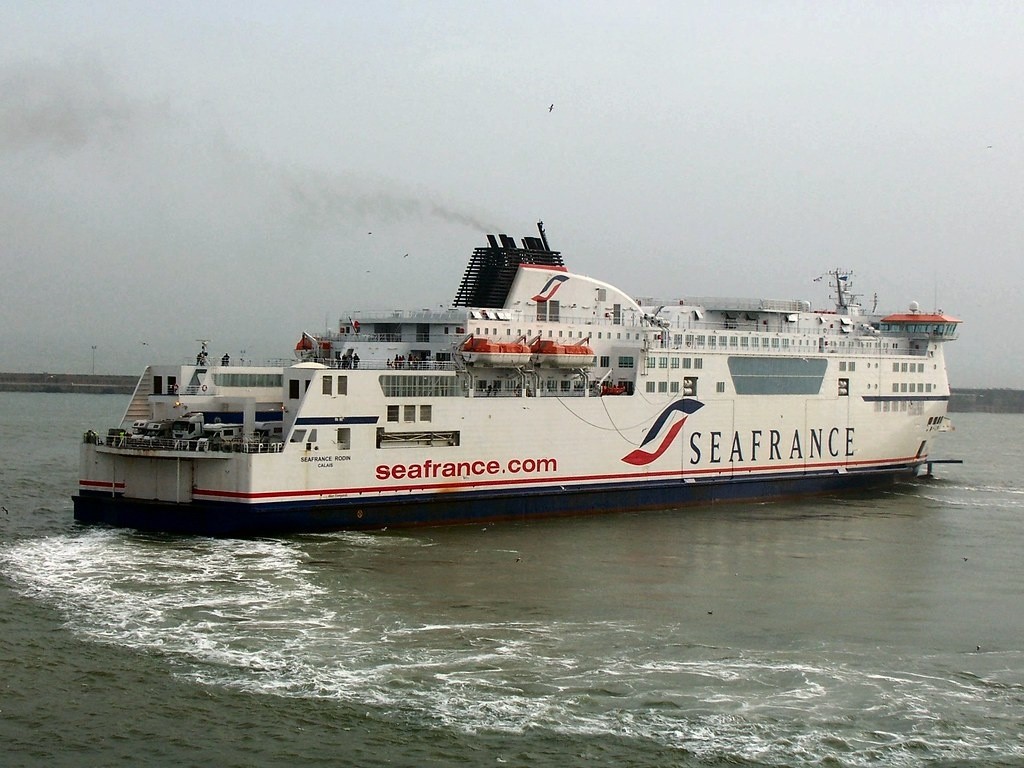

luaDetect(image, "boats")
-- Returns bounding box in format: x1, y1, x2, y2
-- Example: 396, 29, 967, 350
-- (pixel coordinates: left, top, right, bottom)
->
72, 223, 963, 535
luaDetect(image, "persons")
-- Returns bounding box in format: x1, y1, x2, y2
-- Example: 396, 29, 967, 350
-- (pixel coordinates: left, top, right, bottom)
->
221, 353, 230, 366
117, 429, 126, 448
515, 384, 521, 397
336, 353, 360, 370
487, 383, 498, 397
213, 432, 222, 451
387, 353, 419, 370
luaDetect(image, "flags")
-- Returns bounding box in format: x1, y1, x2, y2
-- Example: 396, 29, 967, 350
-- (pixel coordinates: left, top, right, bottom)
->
350, 319, 360, 330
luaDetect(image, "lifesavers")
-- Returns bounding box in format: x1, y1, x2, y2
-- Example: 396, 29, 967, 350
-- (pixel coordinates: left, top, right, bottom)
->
201, 384, 207, 392
173, 384, 179, 390
